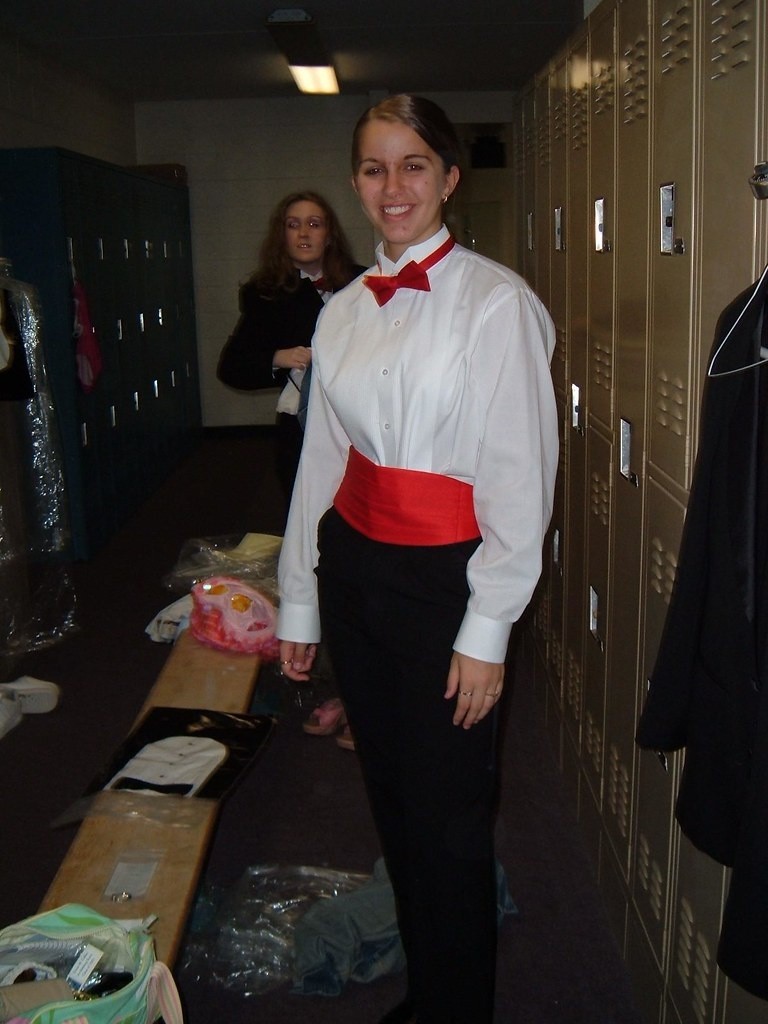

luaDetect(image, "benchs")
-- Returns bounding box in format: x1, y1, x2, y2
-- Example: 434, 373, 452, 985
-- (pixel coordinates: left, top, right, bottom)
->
0, 551, 280, 966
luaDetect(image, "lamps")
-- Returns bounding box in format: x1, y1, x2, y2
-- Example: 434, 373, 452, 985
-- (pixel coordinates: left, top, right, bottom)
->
268, 9, 342, 96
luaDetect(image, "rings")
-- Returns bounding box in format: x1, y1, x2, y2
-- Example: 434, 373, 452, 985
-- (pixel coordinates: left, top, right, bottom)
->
485, 692, 499, 698
280, 657, 294, 665
458, 691, 473, 696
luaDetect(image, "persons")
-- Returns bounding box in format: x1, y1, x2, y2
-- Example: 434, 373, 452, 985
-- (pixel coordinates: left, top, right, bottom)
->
271, 97, 558, 1024
216, 191, 369, 502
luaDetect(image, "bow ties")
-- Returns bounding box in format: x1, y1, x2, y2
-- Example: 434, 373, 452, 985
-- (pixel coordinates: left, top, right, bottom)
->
362, 236, 456, 308
312, 276, 333, 293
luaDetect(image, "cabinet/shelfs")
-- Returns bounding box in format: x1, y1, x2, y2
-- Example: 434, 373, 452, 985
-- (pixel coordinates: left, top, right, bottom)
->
511, 0, 768, 1024
0, 145, 206, 564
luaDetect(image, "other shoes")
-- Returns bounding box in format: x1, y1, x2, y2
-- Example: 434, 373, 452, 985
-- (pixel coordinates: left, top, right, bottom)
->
335, 734, 357, 752
301, 699, 345, 735
